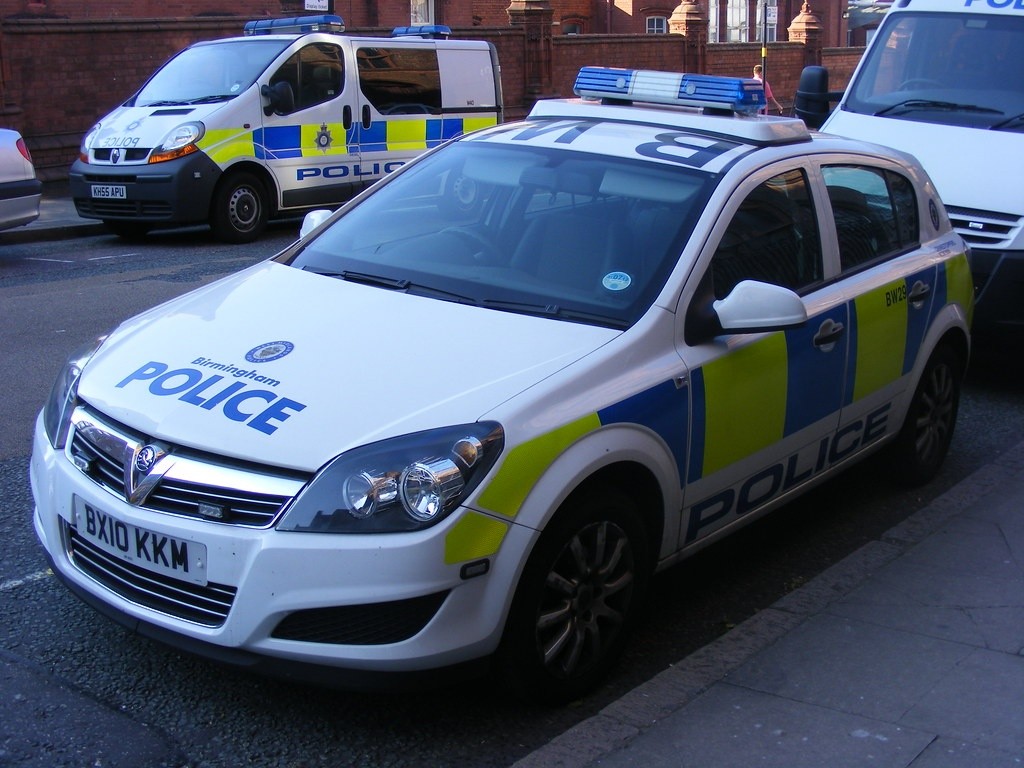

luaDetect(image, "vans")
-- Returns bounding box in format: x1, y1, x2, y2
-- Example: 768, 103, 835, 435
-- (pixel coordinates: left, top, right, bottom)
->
792, 0, 1024, 383
65, 13, 503, 250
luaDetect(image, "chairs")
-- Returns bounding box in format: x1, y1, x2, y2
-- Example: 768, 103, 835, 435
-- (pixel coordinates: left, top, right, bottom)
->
304, 65, 335, 99
633, 201, 692, 256
511, 213, 619, 293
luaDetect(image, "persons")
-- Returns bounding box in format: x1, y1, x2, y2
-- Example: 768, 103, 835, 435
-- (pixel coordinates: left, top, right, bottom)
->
751, 64, 783, 116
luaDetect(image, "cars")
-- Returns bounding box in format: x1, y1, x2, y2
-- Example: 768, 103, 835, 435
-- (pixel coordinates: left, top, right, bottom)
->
0, 121, 44, 231
27, 64, 974, 727
